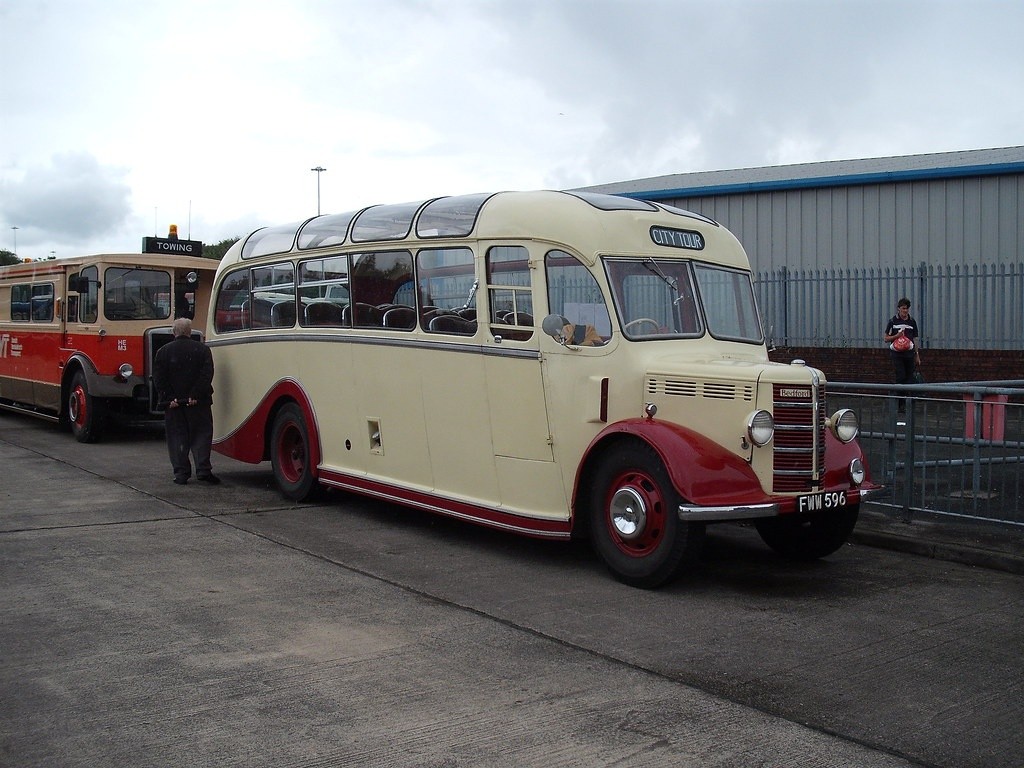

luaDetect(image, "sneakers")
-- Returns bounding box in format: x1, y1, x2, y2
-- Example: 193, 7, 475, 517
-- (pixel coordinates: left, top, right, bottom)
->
173, 478, 187, 485
197, 473, 220, 485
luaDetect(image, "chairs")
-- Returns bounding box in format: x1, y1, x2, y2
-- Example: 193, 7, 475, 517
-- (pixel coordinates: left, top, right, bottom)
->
469, 316, 511, 339
342, 303, 383, 327
452, 307, 468, 313
241, 299, 275, 329
382, 308, 416, 329
305, 301, 342, 326
271, 301, 306, 328
376, 303, 392, 310
424, 309, 460, 330
496, 310, 511, 319
504, 311, 534, 341
428, 315, 472, 333
381, 303, 414, 317
422, 306, 440, 313
458, 308, 477, 321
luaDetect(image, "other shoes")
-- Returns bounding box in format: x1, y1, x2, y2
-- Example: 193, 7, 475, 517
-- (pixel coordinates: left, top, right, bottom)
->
898, 411, 905, 417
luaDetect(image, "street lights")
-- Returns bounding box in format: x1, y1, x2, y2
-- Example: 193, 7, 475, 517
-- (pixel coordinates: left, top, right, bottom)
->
311, 165, 327, 214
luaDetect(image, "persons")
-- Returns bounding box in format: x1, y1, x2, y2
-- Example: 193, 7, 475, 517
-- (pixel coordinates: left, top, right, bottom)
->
885, 299, 921, 414
152, 318, 221, 484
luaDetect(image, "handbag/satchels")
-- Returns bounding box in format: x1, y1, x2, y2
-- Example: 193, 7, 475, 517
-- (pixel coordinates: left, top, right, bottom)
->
912, 363, 928, 402
890, 330, 914, 352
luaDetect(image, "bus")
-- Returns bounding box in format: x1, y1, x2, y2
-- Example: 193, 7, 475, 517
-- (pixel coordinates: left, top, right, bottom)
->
196, 188, 890, 586
0, 235, 219, 445
